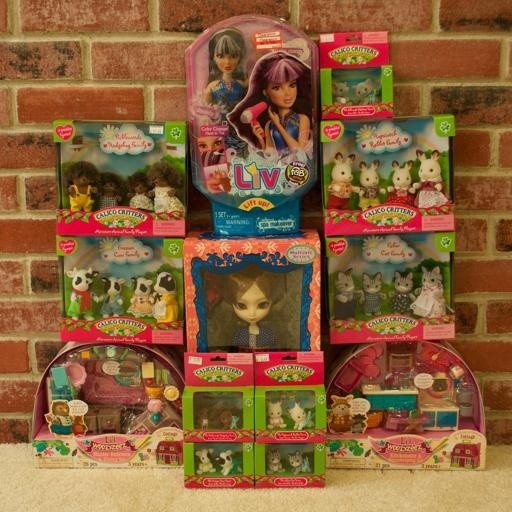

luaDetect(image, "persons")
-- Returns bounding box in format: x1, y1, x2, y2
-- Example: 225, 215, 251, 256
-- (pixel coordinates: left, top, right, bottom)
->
204, 25, 248, 108
223, 49, 311, 152
222, 265, 290, 352
197, 136, 222, 153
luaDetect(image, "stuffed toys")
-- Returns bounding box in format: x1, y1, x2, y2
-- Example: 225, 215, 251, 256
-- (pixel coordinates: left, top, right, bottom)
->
64, 162, 185, 217
